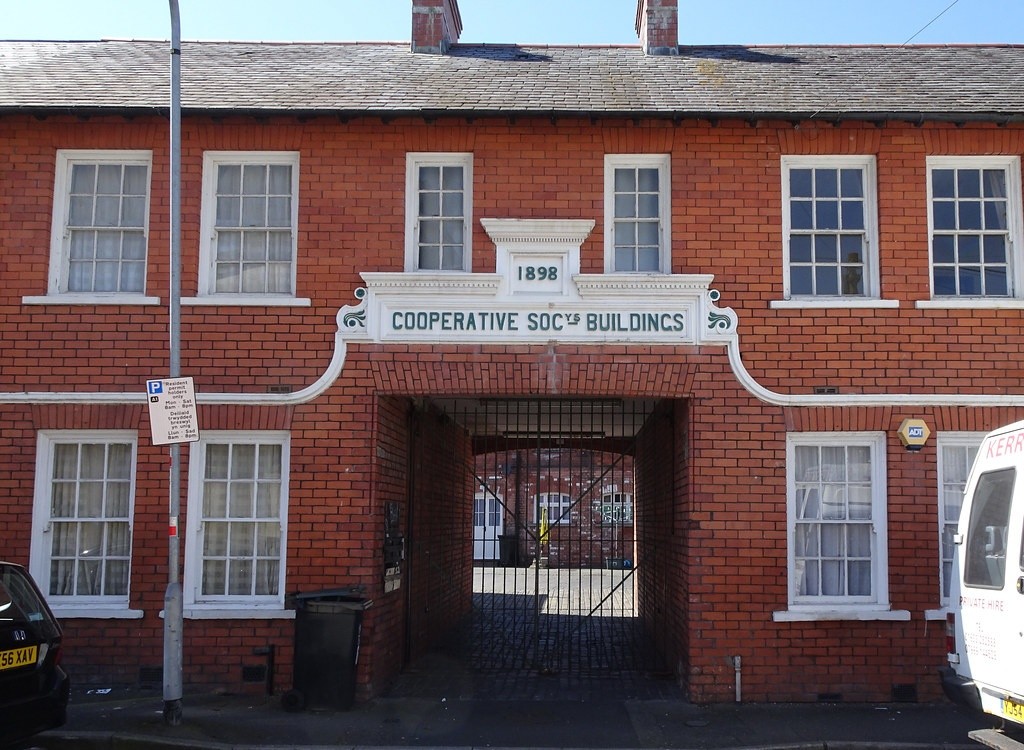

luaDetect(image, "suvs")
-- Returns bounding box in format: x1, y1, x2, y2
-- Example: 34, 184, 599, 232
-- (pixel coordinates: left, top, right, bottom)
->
0, 561, 70, 750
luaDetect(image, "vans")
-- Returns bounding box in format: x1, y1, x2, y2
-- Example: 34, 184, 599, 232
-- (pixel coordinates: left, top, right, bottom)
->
937, 416, 1024, 749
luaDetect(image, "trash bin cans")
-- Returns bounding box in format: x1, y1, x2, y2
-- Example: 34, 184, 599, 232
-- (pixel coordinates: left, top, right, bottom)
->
281, 583, 375, 714
